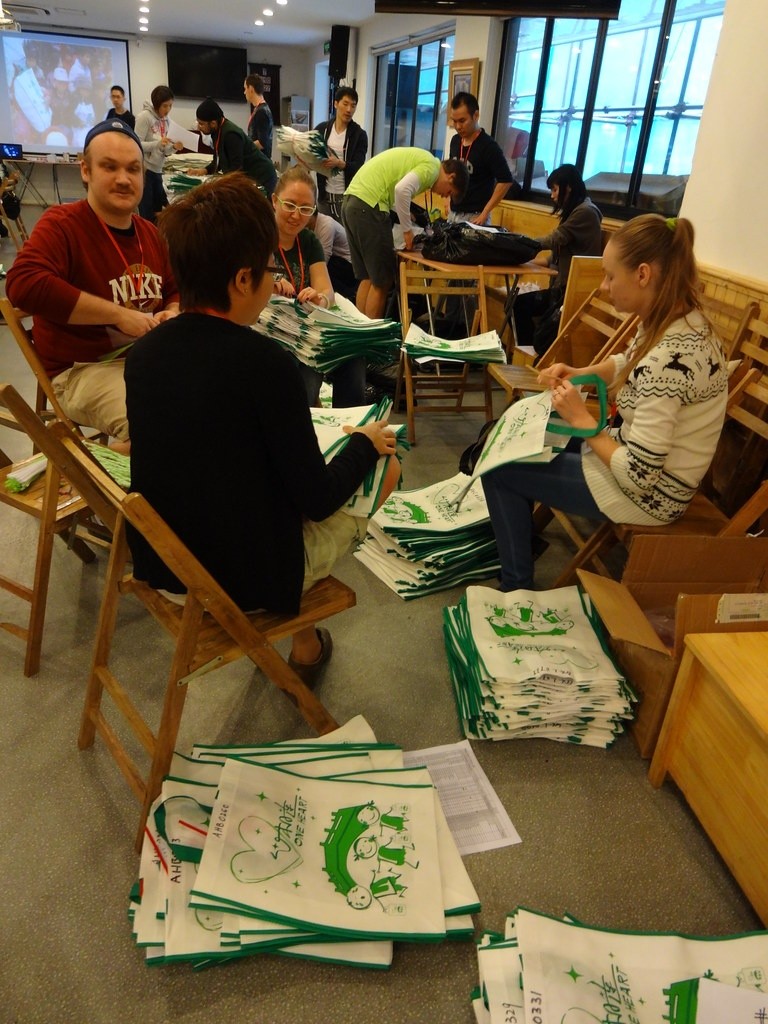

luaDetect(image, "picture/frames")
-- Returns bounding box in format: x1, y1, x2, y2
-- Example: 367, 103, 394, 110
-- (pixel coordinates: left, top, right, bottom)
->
446, 57, 480, 126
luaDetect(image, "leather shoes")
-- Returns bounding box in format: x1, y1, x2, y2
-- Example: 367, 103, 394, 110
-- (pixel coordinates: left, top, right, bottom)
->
289, 625, 333, 692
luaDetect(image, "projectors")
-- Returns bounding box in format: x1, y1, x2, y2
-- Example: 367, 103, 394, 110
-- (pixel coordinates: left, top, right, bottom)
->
0, 19, 21, 33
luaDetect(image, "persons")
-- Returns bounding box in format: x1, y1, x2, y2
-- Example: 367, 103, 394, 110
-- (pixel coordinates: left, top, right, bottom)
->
475, 213, 728, 592
444, 92, 514, 312
268, 166, 368, 409
188, 97, 277, 207
104, 85, 136, 130
133, 85, 183, 222
3, 39, 115, 148
242, 74, 274, 159
295, 85, 369, 228
118, 172, 402, 703
508, 164, 604, 350
304, 209, 361, 300
339, 147, 470, 316
3, 121, 182, 453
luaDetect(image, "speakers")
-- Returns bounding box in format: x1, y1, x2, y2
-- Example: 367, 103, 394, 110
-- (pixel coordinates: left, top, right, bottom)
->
328, 25, 350, 78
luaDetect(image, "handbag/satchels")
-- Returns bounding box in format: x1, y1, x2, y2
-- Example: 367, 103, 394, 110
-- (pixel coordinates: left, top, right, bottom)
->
352, 470, 503, 603
257, 292, 402, 376
310, 382, 411, 518
275, 125, 342, 178
128, 709, 768, 1024
448, 373, 607, 514
166, 171, 215, 194
404, 322, 509, 366
441, 585, 640, 748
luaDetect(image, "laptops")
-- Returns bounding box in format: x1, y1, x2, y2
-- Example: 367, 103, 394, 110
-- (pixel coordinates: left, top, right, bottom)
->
0, 143, 27, 160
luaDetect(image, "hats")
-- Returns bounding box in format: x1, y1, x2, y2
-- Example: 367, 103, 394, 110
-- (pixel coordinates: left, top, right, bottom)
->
196, 97, 223, 122
84, 118, 144, 155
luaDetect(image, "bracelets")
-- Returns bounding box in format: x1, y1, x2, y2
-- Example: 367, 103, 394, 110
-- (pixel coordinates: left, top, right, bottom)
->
319, 293, 330, 309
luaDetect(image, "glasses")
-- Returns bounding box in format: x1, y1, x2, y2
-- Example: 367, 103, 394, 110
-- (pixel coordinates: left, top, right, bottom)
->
231, 265, 286, 282
110, 94, 125, 100
275, 195, 317, 217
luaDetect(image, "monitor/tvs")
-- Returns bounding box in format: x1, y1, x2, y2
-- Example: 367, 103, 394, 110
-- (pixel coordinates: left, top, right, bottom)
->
166, 41, 248, 104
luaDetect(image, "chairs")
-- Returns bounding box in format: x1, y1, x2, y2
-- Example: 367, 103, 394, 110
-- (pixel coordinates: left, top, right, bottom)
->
0, 169, 768, 851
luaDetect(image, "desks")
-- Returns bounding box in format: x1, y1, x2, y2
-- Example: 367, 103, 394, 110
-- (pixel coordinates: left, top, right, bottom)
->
3, 158, 82, 207
394, 247, 557, 394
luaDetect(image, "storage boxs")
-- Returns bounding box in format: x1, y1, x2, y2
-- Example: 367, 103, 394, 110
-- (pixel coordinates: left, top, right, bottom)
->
575, 533, 768, 759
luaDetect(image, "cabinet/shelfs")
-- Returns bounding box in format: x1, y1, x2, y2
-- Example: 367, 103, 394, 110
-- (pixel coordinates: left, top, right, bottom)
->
283, 97, 309, 170
649, 633, 768, 927
